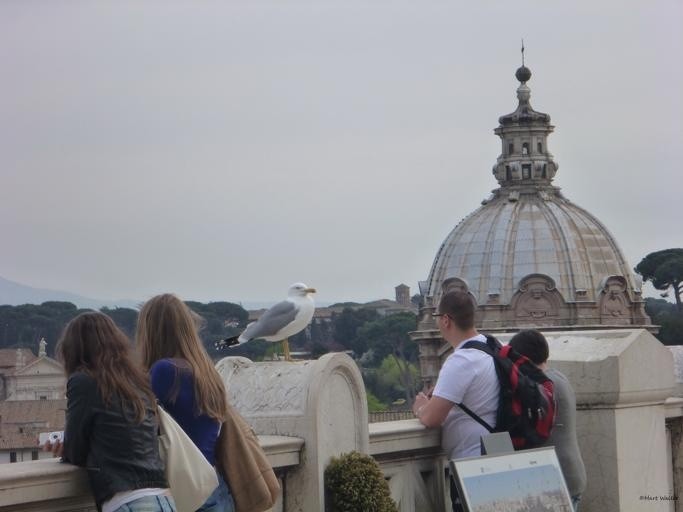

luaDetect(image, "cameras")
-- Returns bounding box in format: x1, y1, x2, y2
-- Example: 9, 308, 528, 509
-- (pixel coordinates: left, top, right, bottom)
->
38, 431, 64, 448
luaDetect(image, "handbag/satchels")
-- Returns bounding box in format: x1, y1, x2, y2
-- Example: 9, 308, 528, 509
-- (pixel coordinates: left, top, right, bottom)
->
218, 404, 281, 512
156, 405, 219, 512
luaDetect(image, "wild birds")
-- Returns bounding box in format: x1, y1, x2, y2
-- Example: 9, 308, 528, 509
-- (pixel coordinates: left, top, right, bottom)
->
213, 281, 318, 361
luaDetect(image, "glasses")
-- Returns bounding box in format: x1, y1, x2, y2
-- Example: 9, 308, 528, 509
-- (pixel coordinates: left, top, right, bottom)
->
431, 312, 453, 321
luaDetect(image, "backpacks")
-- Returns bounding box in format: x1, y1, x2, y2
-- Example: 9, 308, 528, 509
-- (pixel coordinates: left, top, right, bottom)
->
457, 337, 557, 450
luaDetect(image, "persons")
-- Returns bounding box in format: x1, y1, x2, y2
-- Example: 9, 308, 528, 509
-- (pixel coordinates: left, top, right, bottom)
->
134, 293, 238, 512
410, 289, 503, 512
35, 293, 178, 512
506, 327, 587, 512
